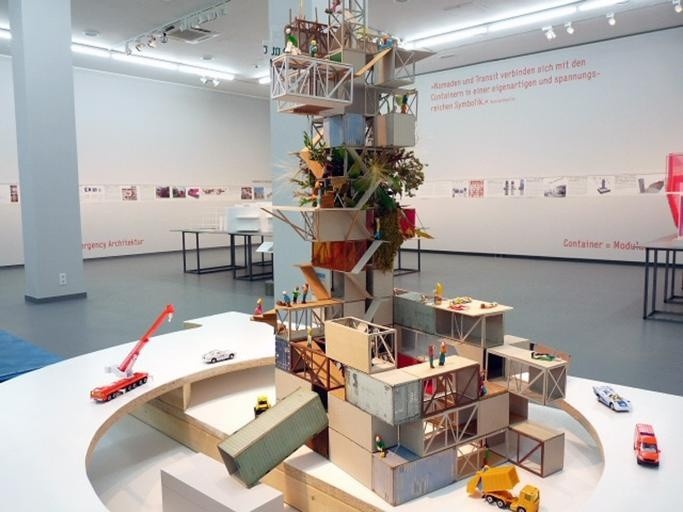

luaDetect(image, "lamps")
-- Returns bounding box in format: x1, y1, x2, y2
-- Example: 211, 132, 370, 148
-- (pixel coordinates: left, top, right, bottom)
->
197, 75, 222, 87
124, 0, 228, 56
539, 1, 682, 40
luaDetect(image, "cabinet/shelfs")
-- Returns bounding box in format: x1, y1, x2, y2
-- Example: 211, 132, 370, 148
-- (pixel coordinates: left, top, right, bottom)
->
217, 0, 569, 512
636, 233, 683, 322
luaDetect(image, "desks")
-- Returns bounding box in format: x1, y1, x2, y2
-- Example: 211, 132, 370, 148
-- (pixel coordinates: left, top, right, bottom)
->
227, 230, 275, 281
169, 227, 248, 275
